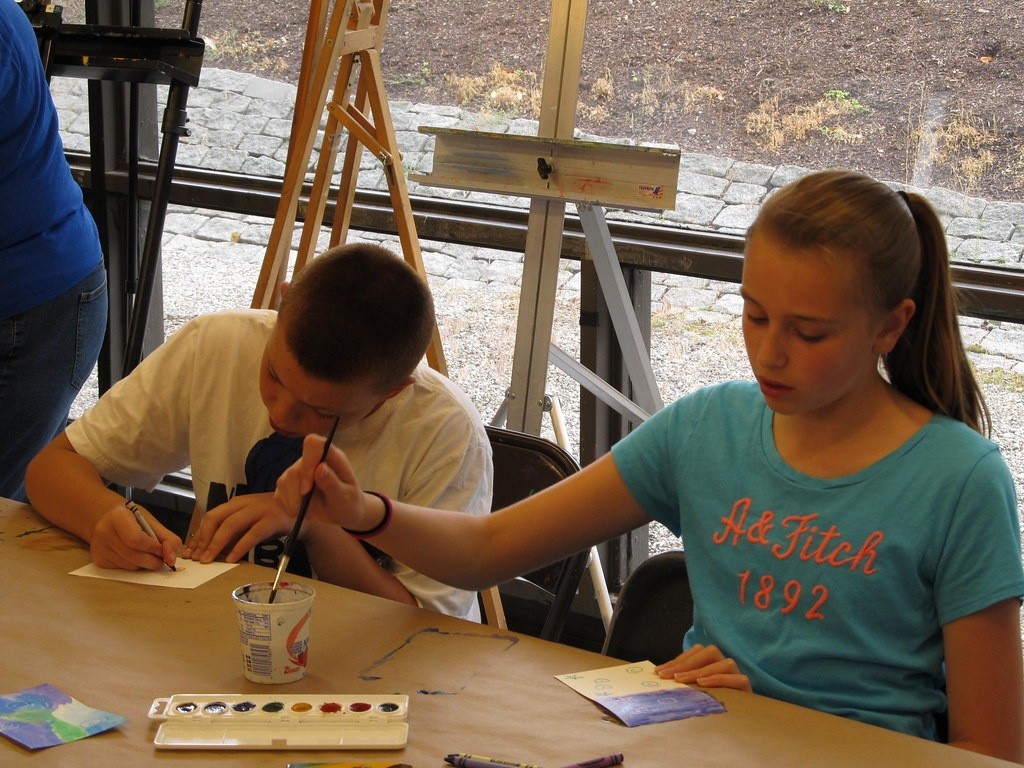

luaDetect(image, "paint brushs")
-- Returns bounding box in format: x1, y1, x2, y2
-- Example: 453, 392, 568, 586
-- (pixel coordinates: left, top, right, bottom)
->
268, 416, 340, 605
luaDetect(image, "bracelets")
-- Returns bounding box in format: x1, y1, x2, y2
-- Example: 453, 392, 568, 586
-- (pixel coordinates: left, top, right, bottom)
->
340, 490, 394, 539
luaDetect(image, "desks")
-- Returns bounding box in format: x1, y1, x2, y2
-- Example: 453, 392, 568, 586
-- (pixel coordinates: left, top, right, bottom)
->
1, 499, 1018, 768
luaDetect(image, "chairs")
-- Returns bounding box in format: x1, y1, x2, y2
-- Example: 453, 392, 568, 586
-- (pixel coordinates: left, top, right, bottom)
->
603, 548, 693, 675
476, 418, 593, 644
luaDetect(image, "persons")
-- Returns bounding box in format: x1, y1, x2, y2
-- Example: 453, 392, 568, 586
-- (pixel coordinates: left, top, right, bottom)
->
0, 0, 115, 497
22, 244, 495, 627
274, 169, 1024, 768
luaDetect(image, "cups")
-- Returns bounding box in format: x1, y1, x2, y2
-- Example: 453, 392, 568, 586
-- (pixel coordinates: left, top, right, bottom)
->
232, 580, 316, 686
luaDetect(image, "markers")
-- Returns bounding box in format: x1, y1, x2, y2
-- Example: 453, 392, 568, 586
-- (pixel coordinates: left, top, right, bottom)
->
125, 500, 177, 572
